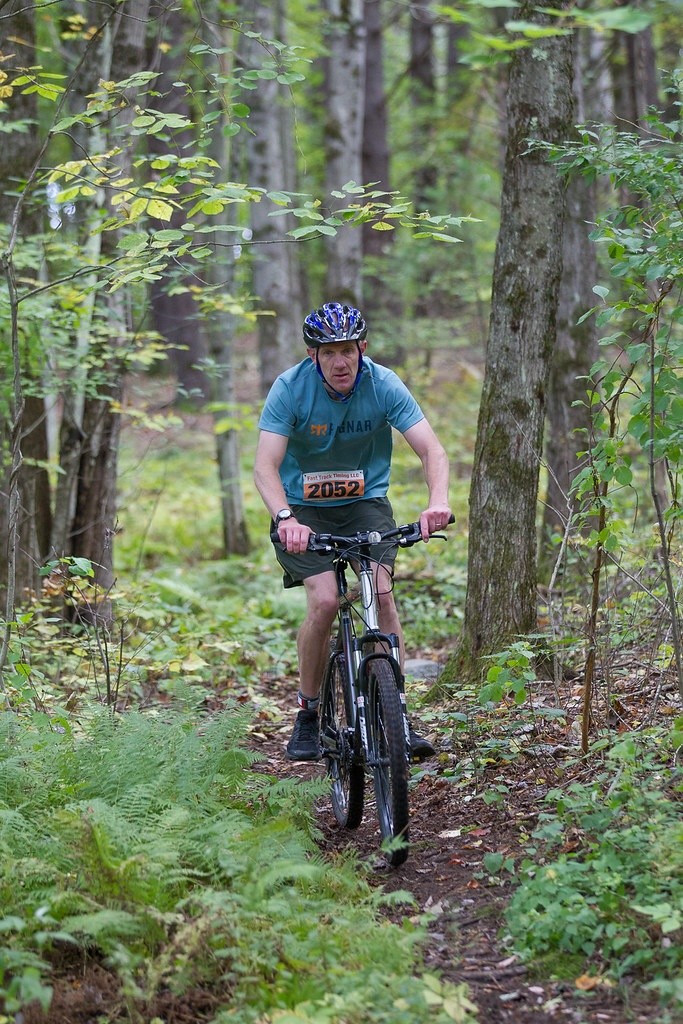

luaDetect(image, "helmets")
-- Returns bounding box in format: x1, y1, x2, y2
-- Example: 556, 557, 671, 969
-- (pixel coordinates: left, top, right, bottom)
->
302, 302, 368, 347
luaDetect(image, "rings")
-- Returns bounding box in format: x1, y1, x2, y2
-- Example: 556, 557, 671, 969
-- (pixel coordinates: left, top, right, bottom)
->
436, 523, 441, 526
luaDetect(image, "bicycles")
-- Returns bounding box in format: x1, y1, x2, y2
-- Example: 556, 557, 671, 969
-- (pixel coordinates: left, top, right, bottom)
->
270, 513, 455, 867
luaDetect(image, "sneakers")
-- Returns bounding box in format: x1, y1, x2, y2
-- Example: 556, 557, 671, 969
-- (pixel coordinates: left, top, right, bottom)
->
407, 721, 436, 758
287, 709, 321, 760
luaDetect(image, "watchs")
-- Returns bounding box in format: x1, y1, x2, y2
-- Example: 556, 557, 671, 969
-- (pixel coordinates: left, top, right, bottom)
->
274, 508, 295, 529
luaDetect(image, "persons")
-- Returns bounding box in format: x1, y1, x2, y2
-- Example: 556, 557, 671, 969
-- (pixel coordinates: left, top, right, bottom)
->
253, 300, 451, 758
